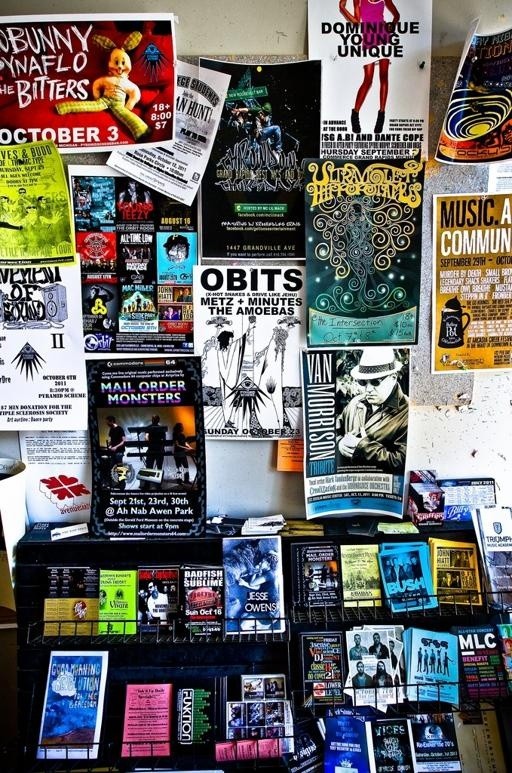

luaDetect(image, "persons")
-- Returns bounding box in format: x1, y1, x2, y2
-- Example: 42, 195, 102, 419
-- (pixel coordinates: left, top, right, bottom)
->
339, 0, 400, 132
83, 284, 193, 335
0, 178, 154, 246
225, 550, 511, 740
227, 100, 283, 158
138, 578, 177, 633
406, 470, 446, 528
336, 347, 408, 474
104, 414, 198, 492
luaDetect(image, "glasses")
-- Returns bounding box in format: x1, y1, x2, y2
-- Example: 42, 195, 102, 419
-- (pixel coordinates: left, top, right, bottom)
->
357, 375, 387, 386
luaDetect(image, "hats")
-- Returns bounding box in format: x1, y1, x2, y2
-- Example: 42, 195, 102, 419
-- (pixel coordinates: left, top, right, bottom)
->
350, 347, 401, 379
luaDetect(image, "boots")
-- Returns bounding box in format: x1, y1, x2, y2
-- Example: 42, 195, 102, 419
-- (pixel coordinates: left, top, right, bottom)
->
374, 110, 384, 132
351, 108, 360, 132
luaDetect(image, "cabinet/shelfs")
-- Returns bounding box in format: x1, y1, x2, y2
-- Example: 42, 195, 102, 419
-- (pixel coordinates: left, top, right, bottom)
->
11, 516, 512, 772
